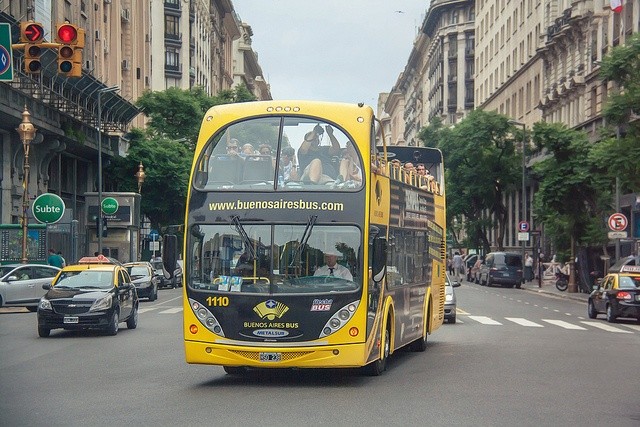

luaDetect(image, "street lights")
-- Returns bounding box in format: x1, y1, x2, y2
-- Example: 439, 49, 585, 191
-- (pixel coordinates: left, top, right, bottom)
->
97, 83, 120, 278
507, 119, 526, 283
15, 103, 38, 263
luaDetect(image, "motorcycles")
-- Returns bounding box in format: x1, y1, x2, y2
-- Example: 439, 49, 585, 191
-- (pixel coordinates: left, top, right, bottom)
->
555, 266, 570, 290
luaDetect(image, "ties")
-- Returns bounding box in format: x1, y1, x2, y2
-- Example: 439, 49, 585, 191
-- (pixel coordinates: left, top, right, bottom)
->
329, 267, 334, 275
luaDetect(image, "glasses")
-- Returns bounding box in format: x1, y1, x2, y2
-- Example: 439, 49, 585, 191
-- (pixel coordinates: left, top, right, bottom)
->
261, 151, 270, 155
225, 146, 238, 151
282, 152, 292, 158
417, 169, 425, 171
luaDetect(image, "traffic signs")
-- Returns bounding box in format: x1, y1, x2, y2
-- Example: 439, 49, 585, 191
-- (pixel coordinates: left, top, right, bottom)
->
134, 160, 145, 257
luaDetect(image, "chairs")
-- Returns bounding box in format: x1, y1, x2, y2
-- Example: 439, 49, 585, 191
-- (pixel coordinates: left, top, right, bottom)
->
241, 155, 274, 182
208, 154, 240, 184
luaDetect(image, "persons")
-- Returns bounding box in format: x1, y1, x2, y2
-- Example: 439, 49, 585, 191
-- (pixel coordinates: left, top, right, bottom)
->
241, 249, 247, 264
98, 273, 111, 285
279, 147, 285, 159
379, 157, 387, 171
268, 145, 277, 158
390, 158, 401, 175
446, 255, 455, 277
240, 143, 254, 161
522, 254, 533, 282
16, 269, 30, 281
256, 244, 265, 255
336, 141, 363, 191
56, 251, 66, 267
259, 143, 271, 157
290, 241, 302, 265
424, 168, 432, 176
59, 271, 78, 286
452, 251, 464, 287
402, 160, 405, 169
297, 123, 337, 192
313, 246, 354, 282
535, 255, 544, 287
413, 166, 416, 172
405, 159, 414, 174
2, 269, 12, 281
279, 146, 297, 182
416, 162, 425, 176
524, 252, 528, 258
46, 248, 65, 269
264, 247, 269, 256
94, 249, 107, 261
370, 145, 379, 171
224, 137, 240, 156
229, 248, 242, 273
226, 126, 232, 146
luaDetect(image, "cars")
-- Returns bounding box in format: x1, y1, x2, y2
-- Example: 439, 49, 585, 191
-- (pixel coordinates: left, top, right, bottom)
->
444, 273, 461, 323
37, 253, 139, 336
0, 264, 62, 311
589, 264, 640, 321
123, 261, 159, 301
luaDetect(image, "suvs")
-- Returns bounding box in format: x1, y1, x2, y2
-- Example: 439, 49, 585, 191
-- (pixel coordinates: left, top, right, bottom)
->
474, 250, 523, 288
149, 260, 182, 289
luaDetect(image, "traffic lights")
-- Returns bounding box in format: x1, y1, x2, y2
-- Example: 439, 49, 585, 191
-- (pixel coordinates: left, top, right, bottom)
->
20, 21, 44, 43
59, 47, 82, 76
25, 42, 41, 73
57, 22, 84, 48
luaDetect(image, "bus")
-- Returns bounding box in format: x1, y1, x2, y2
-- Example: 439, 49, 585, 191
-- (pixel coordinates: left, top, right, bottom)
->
181, 100, 447, 375
